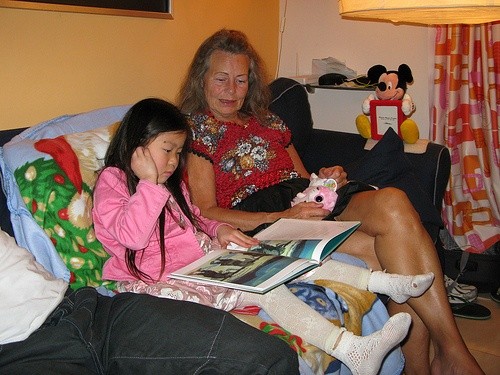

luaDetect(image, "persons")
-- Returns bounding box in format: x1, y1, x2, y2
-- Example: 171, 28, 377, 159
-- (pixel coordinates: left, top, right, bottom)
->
92, 98, 435, 375
0, 229, 301, 375
176, 28, 486, 375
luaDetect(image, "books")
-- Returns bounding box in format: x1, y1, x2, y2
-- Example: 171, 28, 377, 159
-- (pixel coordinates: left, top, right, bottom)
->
167, 217, 362, 295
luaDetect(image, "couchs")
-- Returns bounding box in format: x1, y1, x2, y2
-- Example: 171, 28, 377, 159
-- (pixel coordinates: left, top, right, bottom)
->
0, 78, 451, 375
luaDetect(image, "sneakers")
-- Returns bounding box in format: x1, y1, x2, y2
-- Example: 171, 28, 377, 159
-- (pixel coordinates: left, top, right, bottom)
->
443, 276, 477, 304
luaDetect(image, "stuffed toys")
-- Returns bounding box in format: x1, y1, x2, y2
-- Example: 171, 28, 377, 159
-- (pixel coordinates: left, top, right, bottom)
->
356, 64, 419, 144
291, 173, 338, 212
